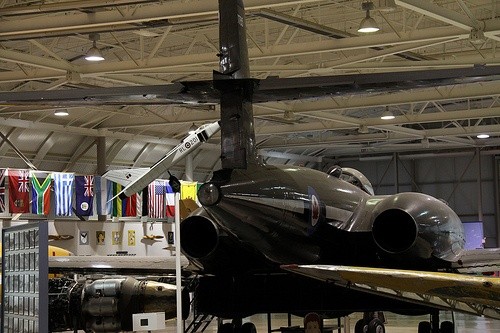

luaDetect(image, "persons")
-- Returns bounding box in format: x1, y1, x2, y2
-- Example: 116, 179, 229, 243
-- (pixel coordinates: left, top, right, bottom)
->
303, 313, 323, 333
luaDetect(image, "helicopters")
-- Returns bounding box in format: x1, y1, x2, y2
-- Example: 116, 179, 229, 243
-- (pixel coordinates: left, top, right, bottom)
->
0, 0, 499, 333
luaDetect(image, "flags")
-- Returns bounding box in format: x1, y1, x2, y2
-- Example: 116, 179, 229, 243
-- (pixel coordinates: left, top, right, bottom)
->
95, 177, 202, 218
32, 172, 51, 215
8, 170, 30, 213
75, 176, 93, 216
0, 169, 6, 213
53, 172, 73, 216
